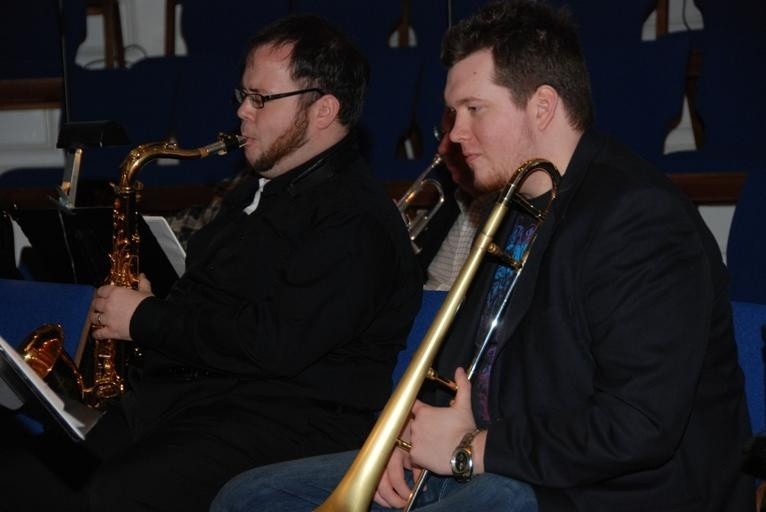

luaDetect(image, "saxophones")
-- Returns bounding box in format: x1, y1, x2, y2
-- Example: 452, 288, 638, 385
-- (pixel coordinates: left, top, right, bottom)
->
11, 132, 246, 410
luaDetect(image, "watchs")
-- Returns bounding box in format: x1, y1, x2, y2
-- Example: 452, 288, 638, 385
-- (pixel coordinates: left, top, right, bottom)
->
450, 427, 485, 484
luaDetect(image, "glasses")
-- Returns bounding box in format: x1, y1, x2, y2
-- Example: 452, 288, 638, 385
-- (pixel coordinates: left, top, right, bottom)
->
233, 87, 325, 108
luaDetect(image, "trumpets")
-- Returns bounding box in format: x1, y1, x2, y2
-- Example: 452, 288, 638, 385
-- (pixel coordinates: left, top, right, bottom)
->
312, 159, 561, 511
392, 155, 447, 254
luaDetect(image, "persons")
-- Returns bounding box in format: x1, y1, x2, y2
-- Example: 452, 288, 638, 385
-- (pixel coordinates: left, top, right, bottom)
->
79, 21, 422, 510
420, 101, 495, 290
205, 2, 754, 511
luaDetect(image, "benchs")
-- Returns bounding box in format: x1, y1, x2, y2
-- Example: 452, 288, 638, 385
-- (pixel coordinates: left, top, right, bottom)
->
0, 278, 766, 512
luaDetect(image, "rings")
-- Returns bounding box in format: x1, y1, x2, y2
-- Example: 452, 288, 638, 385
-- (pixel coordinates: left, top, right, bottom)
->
97, 312, 103, 326
